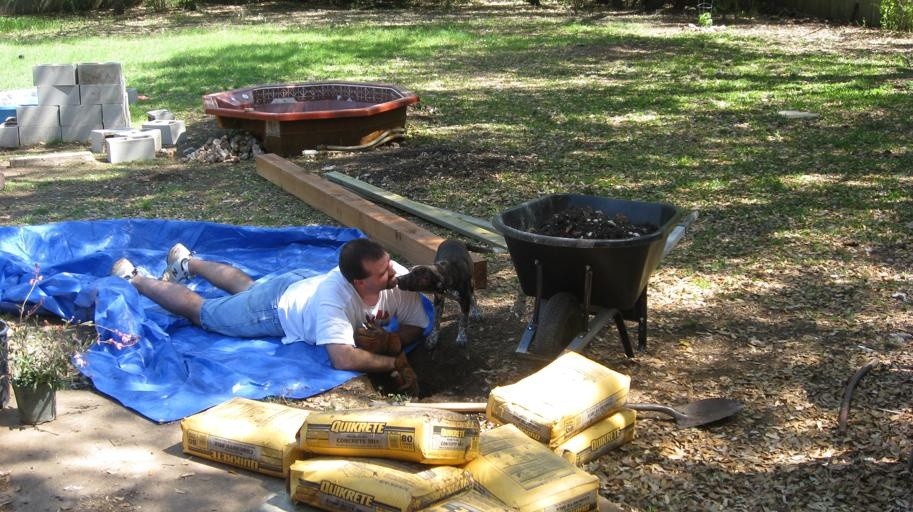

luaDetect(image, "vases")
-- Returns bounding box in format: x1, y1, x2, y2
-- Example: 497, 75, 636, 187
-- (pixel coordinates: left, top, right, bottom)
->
17, 381, 63, 433
0, 322, 10, 405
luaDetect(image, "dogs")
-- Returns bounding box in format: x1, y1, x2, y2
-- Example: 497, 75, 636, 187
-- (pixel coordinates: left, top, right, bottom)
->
396, 238, 494, 352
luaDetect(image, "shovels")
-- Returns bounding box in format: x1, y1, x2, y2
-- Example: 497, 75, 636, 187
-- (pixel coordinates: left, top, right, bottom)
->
402, 397, 746, 431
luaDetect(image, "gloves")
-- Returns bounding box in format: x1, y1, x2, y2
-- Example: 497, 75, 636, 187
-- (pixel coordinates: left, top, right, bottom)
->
355, 320, 402, 357
394, 352, 421, 397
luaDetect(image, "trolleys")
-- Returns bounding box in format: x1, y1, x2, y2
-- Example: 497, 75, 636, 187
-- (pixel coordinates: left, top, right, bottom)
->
488, 195, 696, 365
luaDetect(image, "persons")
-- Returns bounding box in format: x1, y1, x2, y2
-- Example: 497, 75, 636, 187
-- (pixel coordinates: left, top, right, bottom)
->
113, 239, 432, 397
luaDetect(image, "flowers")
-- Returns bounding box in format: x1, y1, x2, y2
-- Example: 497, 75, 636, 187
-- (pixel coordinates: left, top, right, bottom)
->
0, 267, 140, 382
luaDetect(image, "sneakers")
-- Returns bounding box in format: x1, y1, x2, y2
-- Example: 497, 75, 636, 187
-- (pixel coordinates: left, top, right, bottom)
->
110, 255, 139, 285
157, 243, 192, 283
